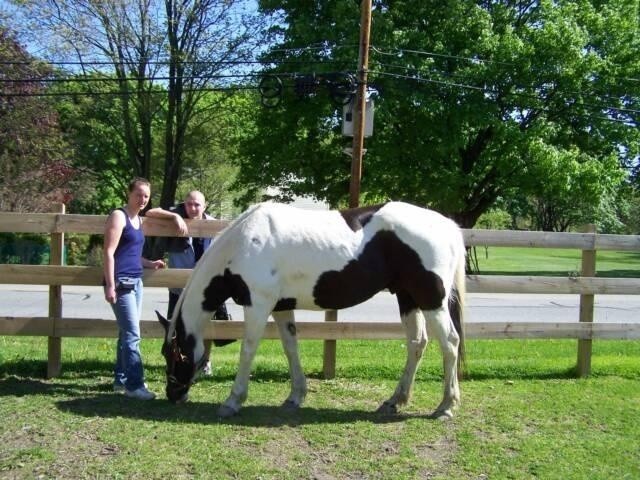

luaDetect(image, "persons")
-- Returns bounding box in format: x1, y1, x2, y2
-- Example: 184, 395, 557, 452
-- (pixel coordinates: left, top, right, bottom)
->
145, 190, 217, 375
103, 176, 166, 401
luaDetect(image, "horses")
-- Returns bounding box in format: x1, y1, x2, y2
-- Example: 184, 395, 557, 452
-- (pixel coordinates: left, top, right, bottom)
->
154, 201, 468, 423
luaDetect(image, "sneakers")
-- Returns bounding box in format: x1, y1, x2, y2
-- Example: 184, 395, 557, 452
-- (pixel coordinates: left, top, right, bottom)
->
202, 361, 212, 376
115, 384, 154, 400
